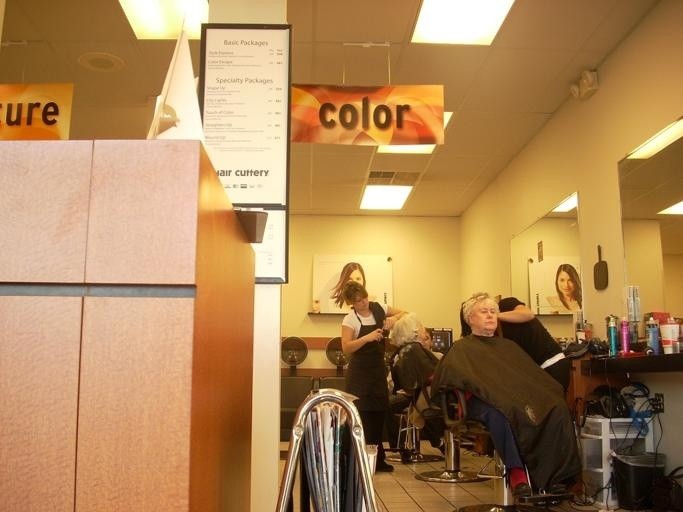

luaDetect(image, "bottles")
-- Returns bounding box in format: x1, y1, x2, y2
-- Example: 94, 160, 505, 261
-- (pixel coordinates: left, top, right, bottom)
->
607, 316, 630, 358
559, 337, 566, 351
575, 320, 591, 344
645, 313, 659, 355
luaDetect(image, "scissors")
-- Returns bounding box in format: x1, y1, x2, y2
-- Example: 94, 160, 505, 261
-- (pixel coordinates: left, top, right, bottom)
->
375, 327, 383, 345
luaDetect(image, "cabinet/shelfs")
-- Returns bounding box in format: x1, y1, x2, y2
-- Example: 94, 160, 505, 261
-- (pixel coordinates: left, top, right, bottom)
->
578, 414, 654, 511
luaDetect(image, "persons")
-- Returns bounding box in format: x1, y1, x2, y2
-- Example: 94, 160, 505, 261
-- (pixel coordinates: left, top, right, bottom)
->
340, 280, 408, 471
543, 260, 583, 314
428, 292, 579, 498
388, 313, 444, 414
412, 325, 443, 361
328, 261, 369, 307
490, 294, 571, 393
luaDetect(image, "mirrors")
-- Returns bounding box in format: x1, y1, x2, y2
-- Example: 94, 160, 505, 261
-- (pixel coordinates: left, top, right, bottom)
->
617, 116, 683, 351
509, 191, 584, 316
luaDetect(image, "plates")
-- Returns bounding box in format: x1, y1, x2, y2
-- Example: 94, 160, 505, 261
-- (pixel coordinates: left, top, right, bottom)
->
280, 336, 308, 366
326, 337, 350, 365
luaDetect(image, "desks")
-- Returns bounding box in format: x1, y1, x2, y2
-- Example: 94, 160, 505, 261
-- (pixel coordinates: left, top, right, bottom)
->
580, 352, 683, 387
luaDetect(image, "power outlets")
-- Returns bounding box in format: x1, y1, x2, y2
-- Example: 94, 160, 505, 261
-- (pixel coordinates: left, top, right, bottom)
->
654, 392, 664, 413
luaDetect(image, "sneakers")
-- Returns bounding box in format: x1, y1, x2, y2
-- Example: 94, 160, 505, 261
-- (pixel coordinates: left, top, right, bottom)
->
376, 461, 394, 472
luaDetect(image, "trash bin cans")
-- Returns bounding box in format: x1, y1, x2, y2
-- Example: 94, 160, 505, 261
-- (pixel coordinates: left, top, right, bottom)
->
611, 451, 664, 510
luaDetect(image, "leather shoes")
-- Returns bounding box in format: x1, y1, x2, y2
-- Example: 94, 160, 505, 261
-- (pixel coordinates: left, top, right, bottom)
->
511, 482, 532, 497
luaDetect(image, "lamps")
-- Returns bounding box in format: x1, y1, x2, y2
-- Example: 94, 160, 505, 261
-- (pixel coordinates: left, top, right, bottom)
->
567, 70, 599, 101
